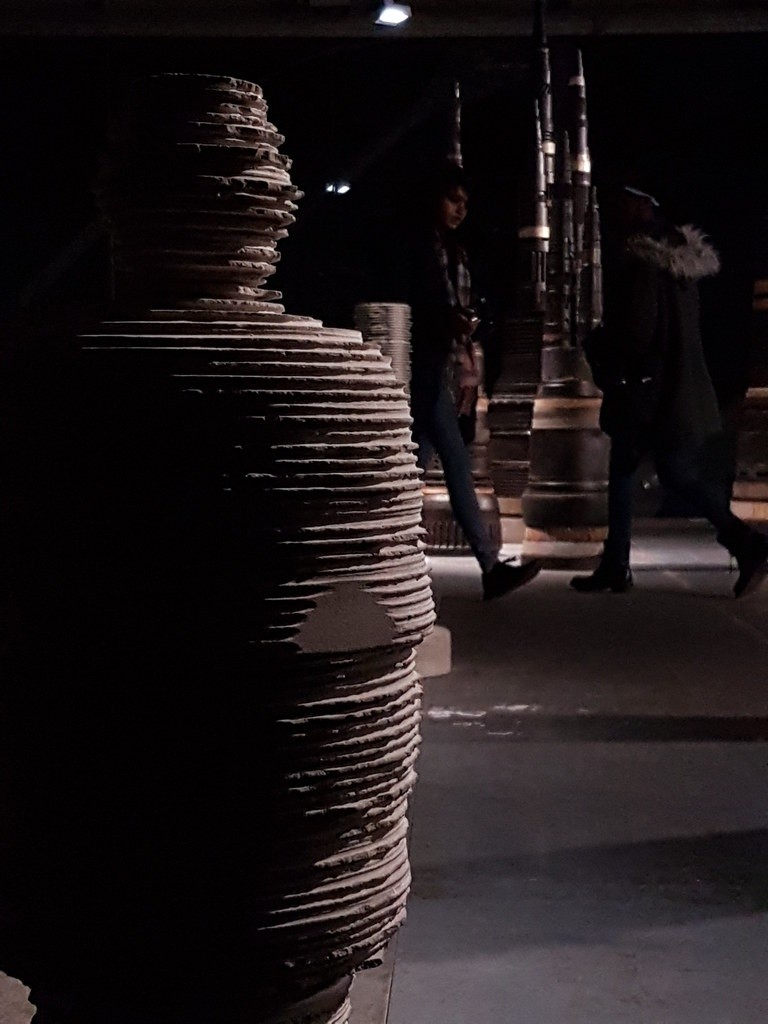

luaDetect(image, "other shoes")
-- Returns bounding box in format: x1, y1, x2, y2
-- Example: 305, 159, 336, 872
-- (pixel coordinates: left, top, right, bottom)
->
483, 559, 544, 599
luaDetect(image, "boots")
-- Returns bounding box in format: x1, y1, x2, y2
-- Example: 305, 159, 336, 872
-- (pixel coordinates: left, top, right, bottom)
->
717, 517, 768, 598
571, 541, 630, 592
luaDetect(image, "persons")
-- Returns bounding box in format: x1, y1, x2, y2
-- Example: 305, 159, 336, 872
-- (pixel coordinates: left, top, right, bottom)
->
570, 174, 768, 597
372, 172, 544, 600
